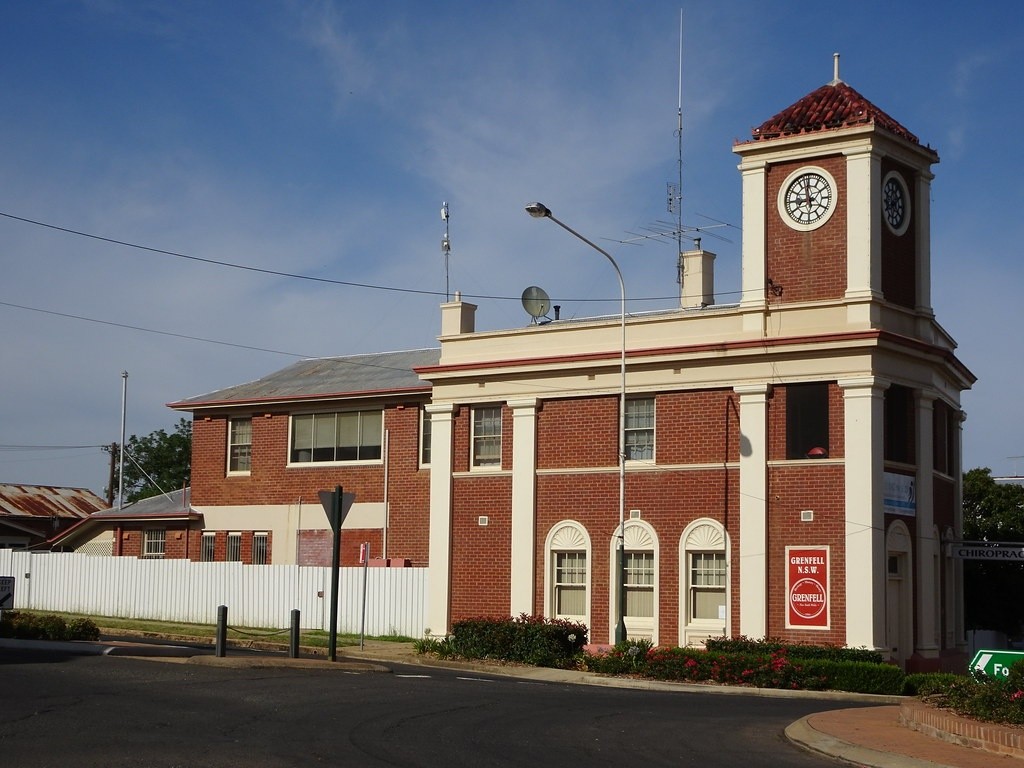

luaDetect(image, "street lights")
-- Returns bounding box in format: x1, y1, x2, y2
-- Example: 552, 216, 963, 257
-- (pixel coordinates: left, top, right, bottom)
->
524, 202, 626, 621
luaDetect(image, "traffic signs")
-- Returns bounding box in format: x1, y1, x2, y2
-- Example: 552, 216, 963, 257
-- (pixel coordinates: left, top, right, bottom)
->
970, 650, 1024, 687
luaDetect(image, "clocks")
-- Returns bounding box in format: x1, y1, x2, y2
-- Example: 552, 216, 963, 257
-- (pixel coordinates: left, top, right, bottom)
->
776, 165, 838, 232
881, 170, 912, 237
